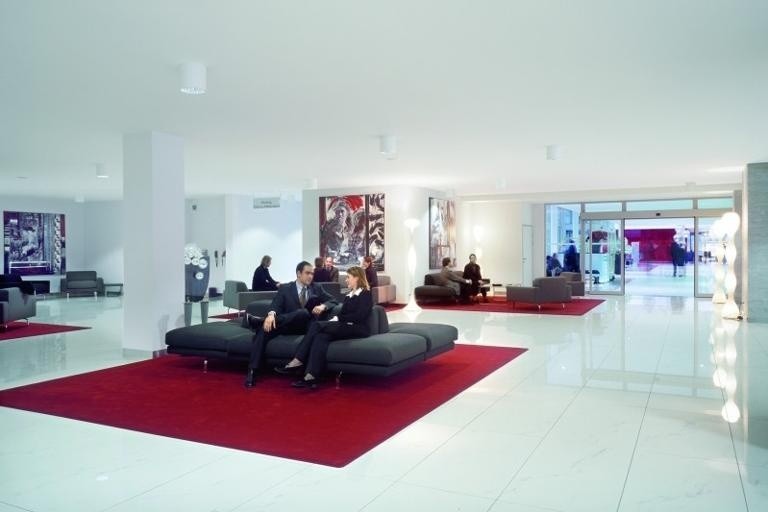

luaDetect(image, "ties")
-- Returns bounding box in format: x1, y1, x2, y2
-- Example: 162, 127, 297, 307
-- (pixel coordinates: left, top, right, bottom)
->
300, 288, 307, 307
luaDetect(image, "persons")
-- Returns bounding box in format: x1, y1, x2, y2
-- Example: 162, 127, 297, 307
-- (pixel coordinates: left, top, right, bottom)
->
324, 256, 339, 282
273, 268, 372, 388
441, 257, 473, 301
551, 252, 567, 273
252, 256, 281, 291
546, 254, 557, 277
313, 255, 332, 282
245, 260, 338, 388
564, 239, 579, 272
362, 256, 378, 287
463, 253, 488, 299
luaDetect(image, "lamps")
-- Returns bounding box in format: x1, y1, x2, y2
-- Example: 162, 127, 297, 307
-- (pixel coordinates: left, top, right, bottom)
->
180, 61, 207, 95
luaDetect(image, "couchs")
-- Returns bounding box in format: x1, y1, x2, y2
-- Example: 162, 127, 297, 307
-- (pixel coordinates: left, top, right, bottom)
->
506, 276, 572, 314
165, 275, 458, 392
65, 271, 103, 293
415, 271, 491, 306
0, 272, 50, 328
532, 271, 584, 296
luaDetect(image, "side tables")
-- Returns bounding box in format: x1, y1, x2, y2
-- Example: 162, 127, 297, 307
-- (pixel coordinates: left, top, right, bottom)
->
102, 283, 123, 297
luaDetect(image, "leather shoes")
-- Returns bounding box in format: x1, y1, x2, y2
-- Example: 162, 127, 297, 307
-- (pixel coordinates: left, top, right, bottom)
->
244, 369, 258, 387
273, 365, 303, 378
290, 379, 318, 389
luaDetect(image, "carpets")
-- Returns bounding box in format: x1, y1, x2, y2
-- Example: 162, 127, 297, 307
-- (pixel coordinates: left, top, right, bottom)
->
417, 295, 607, 316
0, 344, 530, 468
0, 321, 93, 340
